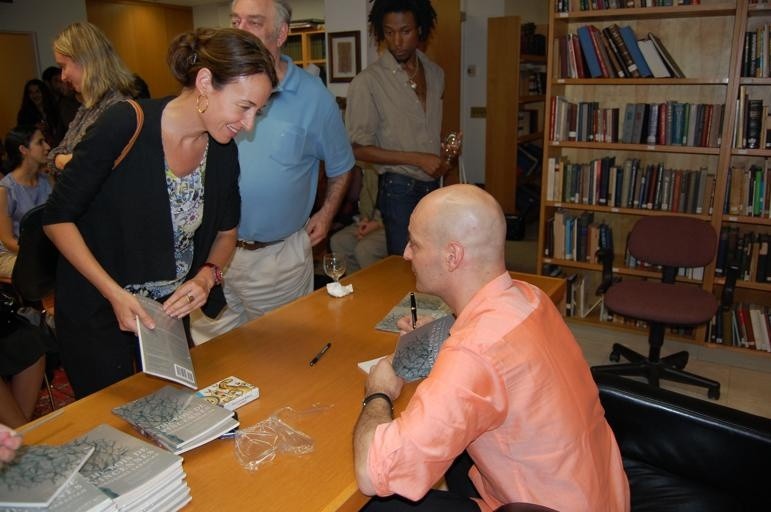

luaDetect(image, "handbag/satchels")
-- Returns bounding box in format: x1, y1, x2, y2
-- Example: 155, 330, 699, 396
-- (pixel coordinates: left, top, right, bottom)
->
11, 97, 145, 304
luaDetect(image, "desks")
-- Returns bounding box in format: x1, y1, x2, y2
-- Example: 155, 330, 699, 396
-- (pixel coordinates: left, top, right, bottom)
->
0, 255, 567, 512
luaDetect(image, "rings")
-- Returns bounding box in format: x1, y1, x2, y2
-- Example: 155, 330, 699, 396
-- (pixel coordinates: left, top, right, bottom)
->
185, 295, 193, 303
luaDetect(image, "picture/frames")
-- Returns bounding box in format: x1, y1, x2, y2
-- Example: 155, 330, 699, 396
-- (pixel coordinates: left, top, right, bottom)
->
327, 29, 361, 84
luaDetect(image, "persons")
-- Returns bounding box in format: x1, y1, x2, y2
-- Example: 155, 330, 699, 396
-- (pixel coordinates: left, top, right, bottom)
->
42, 27, 279, 400
309, 161, 388, 277
0, 312, 45, 463
352, 185, 630, 512
47, 21, 136, 177
345, 1, 445, 257
17, 66, 150, 151
191, 0, 355, 347
1, 125, 57, 327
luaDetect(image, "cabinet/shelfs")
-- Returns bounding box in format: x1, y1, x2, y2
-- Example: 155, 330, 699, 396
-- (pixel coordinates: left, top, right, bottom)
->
486, 14, 545, 238
537, 0, 771, 356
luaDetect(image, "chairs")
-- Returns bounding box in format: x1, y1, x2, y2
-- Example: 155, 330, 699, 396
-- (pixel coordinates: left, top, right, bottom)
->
591, 215, 740, 400
482, 375, 760, 512
316, 166, 364, 240
11, 203, 58, 305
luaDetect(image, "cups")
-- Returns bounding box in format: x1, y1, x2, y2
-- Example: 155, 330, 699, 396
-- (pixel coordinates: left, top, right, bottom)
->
441, 129, 465, 170
323, 253, 345, 282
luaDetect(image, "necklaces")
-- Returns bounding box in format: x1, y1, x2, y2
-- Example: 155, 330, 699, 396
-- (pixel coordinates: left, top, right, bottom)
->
392, 55, 419, 89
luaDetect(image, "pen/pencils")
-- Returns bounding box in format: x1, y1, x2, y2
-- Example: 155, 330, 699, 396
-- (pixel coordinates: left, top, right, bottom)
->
309, 342, 333, 366
409, 292, 418, 330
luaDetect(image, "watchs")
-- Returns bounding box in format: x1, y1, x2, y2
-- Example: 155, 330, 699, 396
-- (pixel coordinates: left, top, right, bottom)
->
198, 262, 225, 285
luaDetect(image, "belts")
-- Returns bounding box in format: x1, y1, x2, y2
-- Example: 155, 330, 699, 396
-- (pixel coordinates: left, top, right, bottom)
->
235, 239, 284, 250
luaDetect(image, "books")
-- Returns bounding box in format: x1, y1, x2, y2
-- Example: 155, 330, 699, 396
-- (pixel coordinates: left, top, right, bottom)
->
517, 58, 547, 137
715, 226, 771, 285
554, 24, 686, 78
708, 293, 771, 354
740, 24, 771, 78
731, 86, 771, 149
554, 0, 703, 12
0, 445, 120, 512
542, 209, 614, 262
543, 265, 605, 322
63, 423, 192, 512
678, 267, 705, 280
281, 18, 326, 62
391, 315, 454, 384
549, 95, 725, 147
722, 156, 771, 218
374, 290, 454, 333
111, 385, 241, 455
133, 293, 198, 390
546, 155, 717, 215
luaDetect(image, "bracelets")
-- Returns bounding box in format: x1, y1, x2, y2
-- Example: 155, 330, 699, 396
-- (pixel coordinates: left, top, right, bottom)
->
363, 393, 395, 418
